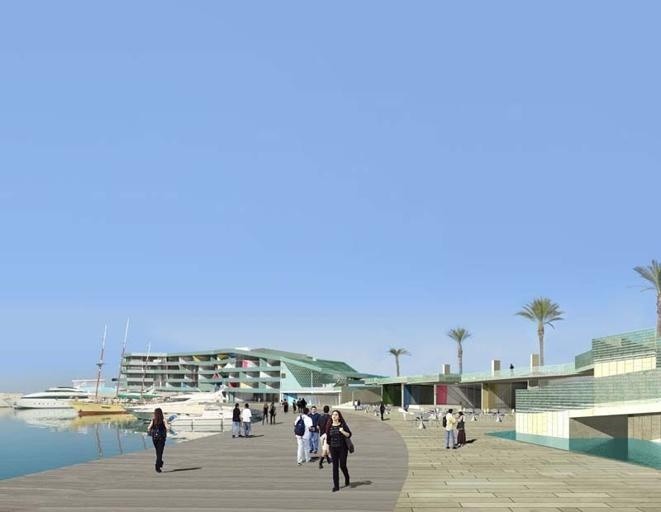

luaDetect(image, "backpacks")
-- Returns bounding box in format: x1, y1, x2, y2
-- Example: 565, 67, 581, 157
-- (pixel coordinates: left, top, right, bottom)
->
294, 415, 304, 435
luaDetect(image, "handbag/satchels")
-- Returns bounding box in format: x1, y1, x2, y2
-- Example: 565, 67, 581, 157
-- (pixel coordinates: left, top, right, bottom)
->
153, 425, 165, 440
345, 438, 353, 453
457, 423, 464, 430
443, 417, 446, 427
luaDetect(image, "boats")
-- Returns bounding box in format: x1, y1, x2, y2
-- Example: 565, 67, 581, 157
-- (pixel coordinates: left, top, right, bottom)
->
14, 381, 91, 409
10, 407, 77, 434
124, 386, 225, 423
165, 410, 237, 426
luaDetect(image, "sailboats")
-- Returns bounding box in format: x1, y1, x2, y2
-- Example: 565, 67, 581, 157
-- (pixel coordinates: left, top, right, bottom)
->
70, 416, 146, 460
71, 315, 152, 415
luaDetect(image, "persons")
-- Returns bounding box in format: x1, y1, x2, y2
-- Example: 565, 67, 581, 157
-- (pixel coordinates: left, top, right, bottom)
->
317, 406, 333, 468
294, 408, 313, 465
240, 404, 253, 436
354, 400, 357, 410
232, 403, 242, 437
262, 398, 307, 425
446, 409, 457, 449
325, 410, 351, 491
147, 408, 168, 472
357, 400, 360, 410
380, 403, 385, 420
457, 412, 466, 447
509, 364, 514, 376
308, 406, 321, 453
